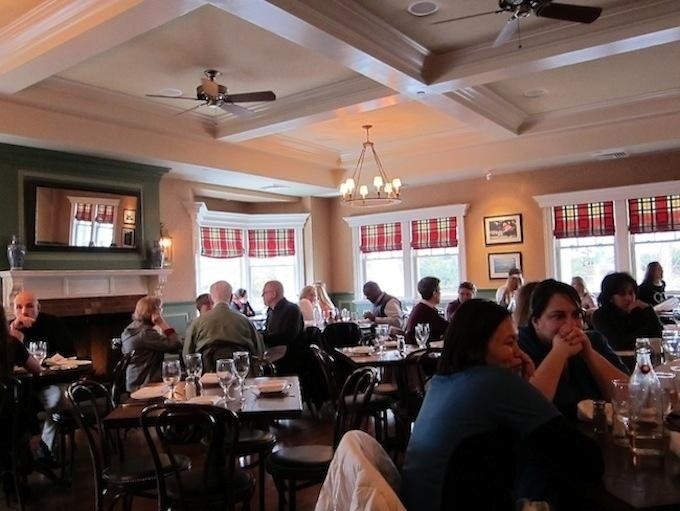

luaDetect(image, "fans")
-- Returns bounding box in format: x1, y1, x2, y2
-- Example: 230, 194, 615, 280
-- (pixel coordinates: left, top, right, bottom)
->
145, 69, 275, 120
431, 0, 603, 50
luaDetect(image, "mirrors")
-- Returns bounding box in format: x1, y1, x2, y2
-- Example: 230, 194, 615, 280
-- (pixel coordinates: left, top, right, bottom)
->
29, 179, 142, 252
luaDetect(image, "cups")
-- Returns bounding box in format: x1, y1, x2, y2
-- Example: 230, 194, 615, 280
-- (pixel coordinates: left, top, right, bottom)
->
184, 374, 205, 401
611, 330, 680, 446
29, 342, 47, 371
363, 310, 370, 320
673, 308, 680, 329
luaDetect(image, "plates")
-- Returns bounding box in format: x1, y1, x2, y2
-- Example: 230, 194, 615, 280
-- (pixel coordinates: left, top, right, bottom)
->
200, 373, 220, 386
131, 387, 169, 400
352, 348, 370, 355
257, 380, 288, 394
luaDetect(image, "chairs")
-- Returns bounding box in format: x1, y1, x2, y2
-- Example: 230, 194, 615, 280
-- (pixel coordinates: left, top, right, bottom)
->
0, 315, 680, 511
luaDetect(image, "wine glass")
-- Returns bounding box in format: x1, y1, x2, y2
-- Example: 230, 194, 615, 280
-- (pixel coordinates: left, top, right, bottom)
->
216, 359, 235, 403
415, 324, 431, 350
374, 325, 389, 355
163, 361, 182, 404
187, 353, 202, 380
233, 352, 251, 392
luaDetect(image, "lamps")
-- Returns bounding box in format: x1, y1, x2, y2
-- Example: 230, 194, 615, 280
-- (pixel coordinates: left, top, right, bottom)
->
340, 125, 402, 209
160, 222, 173, 268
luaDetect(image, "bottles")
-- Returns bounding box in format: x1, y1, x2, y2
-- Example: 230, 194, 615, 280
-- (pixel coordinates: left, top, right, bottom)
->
313, 299, 358, 329
628, 338, 665, 459
396, 336, 405, 352
591, 400, 610, 435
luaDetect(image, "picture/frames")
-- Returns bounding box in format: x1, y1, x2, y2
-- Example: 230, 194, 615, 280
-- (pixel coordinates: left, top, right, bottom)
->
484, 214, 523, 245
488, 252, 522, 279
123, 209, 136, 224
121, 226, 135, 249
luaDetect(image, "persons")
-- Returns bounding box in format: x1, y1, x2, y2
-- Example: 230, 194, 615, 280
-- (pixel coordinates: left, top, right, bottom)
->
298, 281, 335, 320
496, 269, 525, 313
119, 295, 182, 394
385, 298, 613, 511
404, 277, 449, 344
262, 281, 304, 367
570, 277, 599, 310
0, 301, 45, 497
447, 282, 477, 318
507, 279, 636, 413
638, 262, 670, 324
181, 281, 265, 368
362, 281, 403, 329
8, 290, 65, 481
